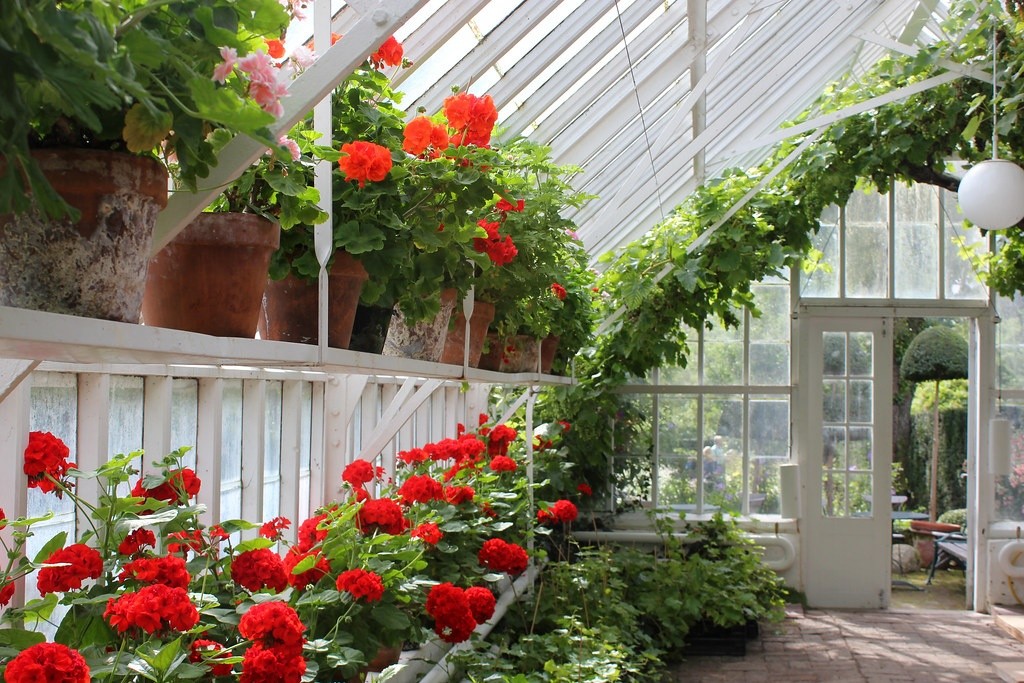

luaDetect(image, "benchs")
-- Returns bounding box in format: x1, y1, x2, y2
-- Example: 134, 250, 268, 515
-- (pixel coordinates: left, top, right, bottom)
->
926, 518, 989, 587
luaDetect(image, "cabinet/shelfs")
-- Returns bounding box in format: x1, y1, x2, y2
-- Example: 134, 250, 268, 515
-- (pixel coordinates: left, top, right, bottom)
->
0, 307, 578, 683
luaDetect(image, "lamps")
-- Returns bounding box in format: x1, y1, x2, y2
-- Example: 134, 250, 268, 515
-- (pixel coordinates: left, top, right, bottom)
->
780, 316, 802, 519
955, 13, 1024, 230
988, 324, 1012, 478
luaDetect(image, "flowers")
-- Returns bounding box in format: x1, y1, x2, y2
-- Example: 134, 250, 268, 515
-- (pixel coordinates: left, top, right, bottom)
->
0, 1, 617, 314
0, 400, 611, 683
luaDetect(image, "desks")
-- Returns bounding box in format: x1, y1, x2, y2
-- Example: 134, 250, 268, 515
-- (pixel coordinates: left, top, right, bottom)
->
851, 512, 928, 590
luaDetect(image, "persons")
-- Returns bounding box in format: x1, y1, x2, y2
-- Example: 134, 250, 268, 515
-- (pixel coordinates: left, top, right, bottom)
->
704, 436, 723, 492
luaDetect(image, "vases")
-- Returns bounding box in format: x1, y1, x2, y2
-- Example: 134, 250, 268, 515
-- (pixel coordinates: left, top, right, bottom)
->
0, 151, 180, 323
145, 207, 284, 338
536, 337, 564, 373
910, 521, 962, 569
441, 297, 504, 364
337, 266, 411, 357
386, 280, 458, 360
486, 316, 533, 377
550, 348, 571, 376
504, 336, 544, 373
363, 635, 405, 673
249, 243, 365, 348
